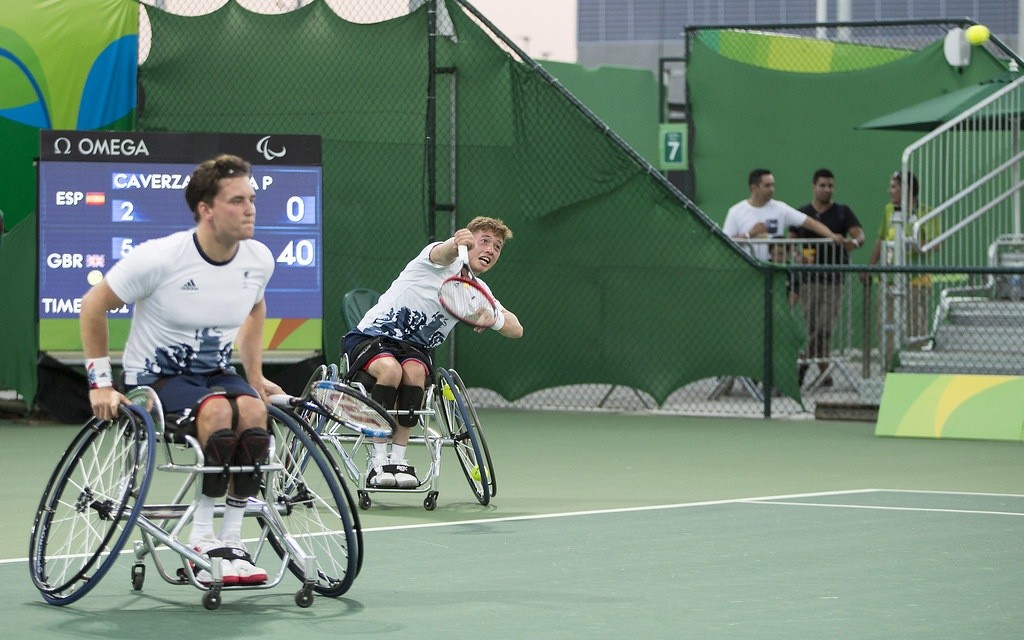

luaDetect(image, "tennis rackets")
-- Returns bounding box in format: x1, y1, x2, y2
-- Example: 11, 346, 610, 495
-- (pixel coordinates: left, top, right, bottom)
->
269, 378, 398, 440
437, 243, 500, 328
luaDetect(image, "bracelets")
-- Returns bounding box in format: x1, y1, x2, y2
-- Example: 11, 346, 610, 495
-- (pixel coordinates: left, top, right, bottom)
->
487, 308, 505, 330
744, 230, 751, 238
84, 356, 113, 390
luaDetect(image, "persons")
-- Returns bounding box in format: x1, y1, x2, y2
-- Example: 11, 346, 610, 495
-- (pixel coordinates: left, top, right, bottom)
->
722, 169, 864, 389
340, 217, 522, 487
860, 171, 944, 373
80, 155, 287, 585
851, 239, 859, 248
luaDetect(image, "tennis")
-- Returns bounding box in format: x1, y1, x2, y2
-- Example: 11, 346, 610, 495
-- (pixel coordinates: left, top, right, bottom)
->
964, 24, 991, 46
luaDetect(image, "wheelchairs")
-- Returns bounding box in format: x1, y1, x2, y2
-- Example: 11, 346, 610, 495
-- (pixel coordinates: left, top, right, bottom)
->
276, 355, 499, 508
23, 381, 373, 616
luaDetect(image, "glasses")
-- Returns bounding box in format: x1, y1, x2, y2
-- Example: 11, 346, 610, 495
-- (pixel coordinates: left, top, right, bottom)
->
890, 170, 901, 180
220, 167, 253, 175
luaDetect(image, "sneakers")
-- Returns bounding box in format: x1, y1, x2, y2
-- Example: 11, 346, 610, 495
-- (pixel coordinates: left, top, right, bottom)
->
390, 459, 419, 487
365, 457, 396, 486
217, 536, 269, 583
184, 538, 240, 583
